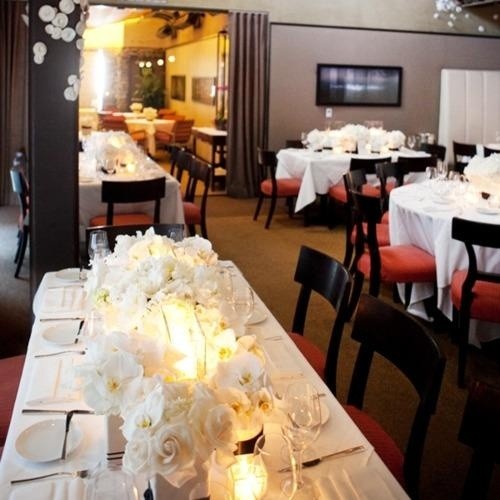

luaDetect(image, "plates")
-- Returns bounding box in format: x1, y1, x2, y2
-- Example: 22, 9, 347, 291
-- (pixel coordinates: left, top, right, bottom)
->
14, 418, 83, 463
54, 267, 89, 282
246, 307, 267, 325
320, 403, 329, 424
42, 323, 82, 345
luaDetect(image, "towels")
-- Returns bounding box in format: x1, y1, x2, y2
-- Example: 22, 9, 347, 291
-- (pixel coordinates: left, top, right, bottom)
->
44, 363, 84, 405
285, 466, 394, 500
27, 357, 62, 405
7, 477, 87, 500
41, 287, 86, 312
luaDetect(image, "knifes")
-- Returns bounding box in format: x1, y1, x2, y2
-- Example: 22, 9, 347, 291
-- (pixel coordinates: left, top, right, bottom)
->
20, 408, 105, 416
75, 320, 85, 343
278, 445, 368, 475
61, 412, 75, 460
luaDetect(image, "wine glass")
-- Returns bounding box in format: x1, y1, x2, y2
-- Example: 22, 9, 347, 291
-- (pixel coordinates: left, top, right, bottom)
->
300, 118, 417, 156
423, 158, 462, 200
281, 379, 323, 499
102, 131, 148, 180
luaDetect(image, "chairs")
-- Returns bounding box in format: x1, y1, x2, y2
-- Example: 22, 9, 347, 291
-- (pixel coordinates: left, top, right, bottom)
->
284, 242, 352, 397
7, 99, 234, 275
249, 133, 500, 388
337, 289, 446, 500
456, 382, 499, 500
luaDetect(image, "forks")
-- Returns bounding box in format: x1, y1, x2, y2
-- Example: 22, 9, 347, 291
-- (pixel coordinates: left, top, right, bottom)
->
34, 348, 90, 359
10, 461, 102, 487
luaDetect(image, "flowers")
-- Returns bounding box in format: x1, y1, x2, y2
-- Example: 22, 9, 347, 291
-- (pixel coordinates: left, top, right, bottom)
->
82, 225, 275, 500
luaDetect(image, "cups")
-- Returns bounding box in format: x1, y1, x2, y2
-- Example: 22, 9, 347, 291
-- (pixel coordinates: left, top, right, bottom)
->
168, 229, 186, 243
254, 435, 294, 500
232, 286, 256, 323
88, 229, 109, 259
216, 265, 233, 301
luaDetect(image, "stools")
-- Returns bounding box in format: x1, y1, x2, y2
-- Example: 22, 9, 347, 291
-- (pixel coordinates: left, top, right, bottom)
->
0, 354, 28, 445
